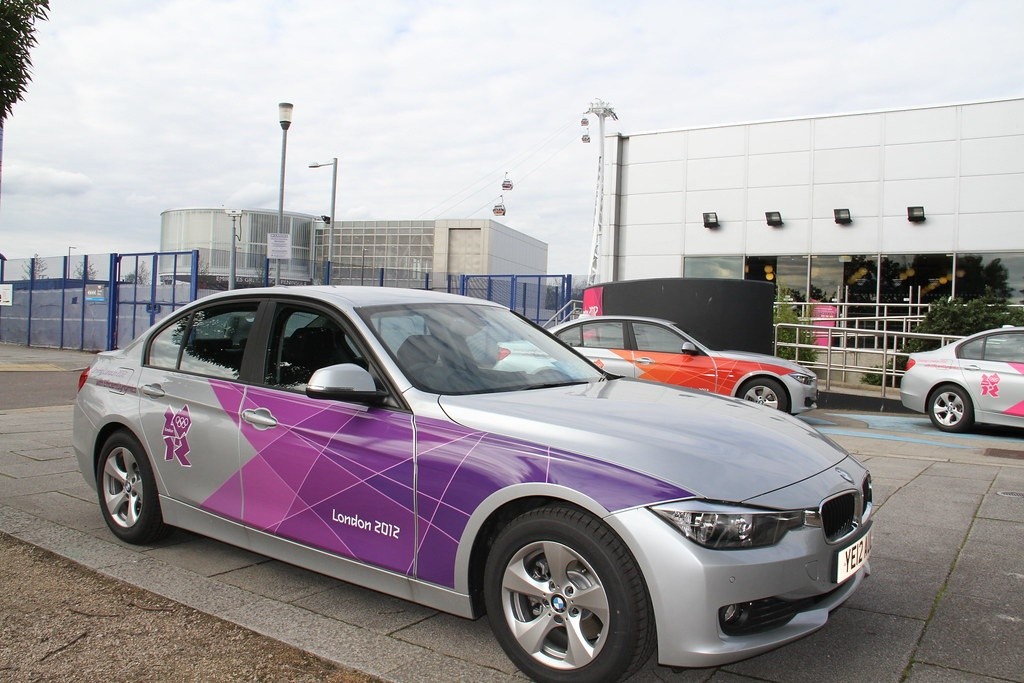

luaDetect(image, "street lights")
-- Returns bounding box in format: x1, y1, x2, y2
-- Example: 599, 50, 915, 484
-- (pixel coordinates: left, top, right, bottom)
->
68, 246, 77, 279
275, 99, 295, 287
308, 158, 338, 285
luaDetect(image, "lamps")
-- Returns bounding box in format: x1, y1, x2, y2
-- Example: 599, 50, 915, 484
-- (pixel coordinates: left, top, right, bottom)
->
907, 206, 926, 223
834, 208, 852, 225
765, 212, 784, 227
703, 212, 721, 229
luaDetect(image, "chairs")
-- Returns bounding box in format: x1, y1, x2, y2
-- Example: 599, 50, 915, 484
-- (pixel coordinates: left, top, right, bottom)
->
283, 327, 335, 385
397, 335, 439, 375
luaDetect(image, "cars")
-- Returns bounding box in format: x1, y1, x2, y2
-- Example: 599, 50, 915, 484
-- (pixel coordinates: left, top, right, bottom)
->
72, 286, 874, 683
901, 324, 1024, 434
495, 315, 820, 415
